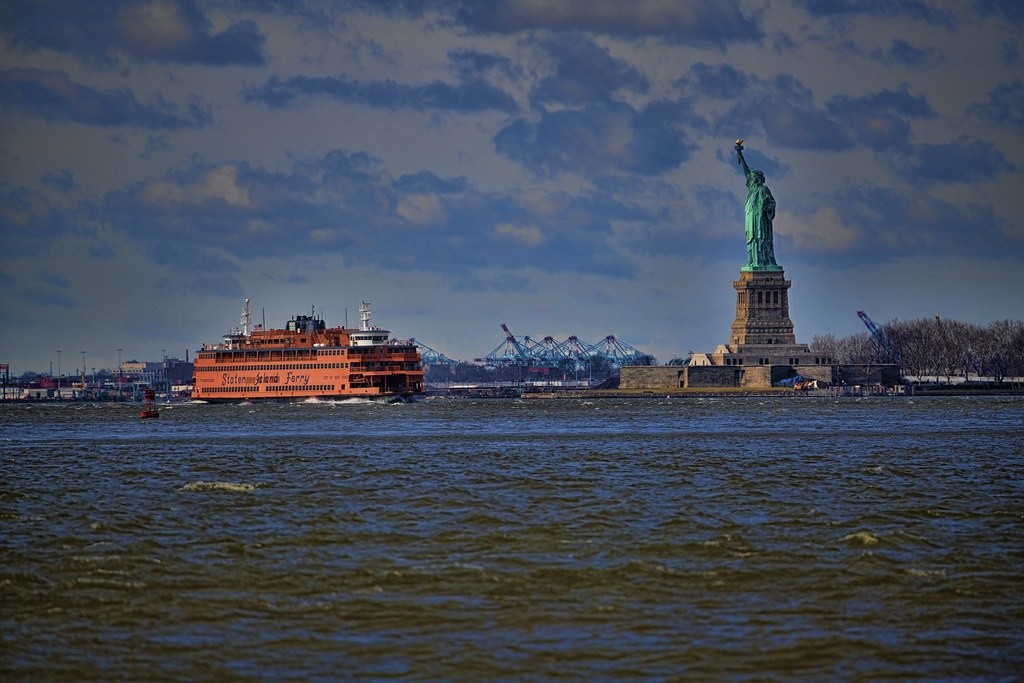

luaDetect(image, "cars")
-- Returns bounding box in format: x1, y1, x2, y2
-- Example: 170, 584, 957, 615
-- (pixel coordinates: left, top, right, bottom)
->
794, 383, 810, 390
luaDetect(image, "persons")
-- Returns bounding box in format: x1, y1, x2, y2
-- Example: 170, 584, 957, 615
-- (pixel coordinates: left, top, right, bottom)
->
736, 151, 777, 269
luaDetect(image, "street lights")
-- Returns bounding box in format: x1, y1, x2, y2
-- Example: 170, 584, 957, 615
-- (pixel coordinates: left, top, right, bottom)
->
80, 351, 87, 393
117, 349, 123, 396
162, 350, 165, 388
57, 350, 62, 398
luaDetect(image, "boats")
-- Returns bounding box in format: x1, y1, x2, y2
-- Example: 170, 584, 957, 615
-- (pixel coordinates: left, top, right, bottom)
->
141, 390, 160, 420
189, 297, 425, 404
